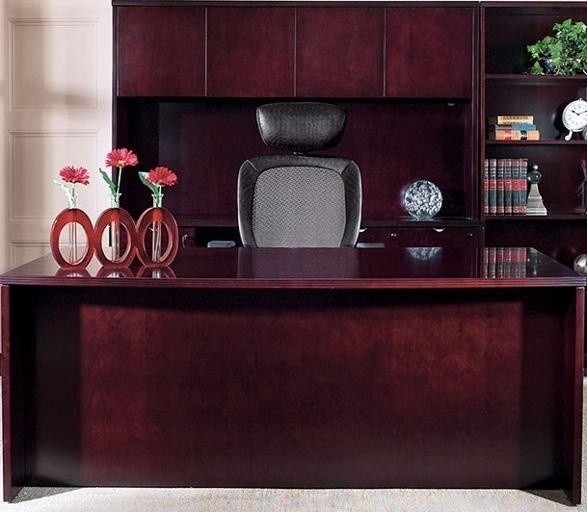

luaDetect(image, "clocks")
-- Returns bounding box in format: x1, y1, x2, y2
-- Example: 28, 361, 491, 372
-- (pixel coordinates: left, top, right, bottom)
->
559, 95, 586, 141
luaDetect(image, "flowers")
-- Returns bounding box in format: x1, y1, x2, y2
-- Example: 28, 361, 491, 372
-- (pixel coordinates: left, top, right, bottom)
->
58, 148, 179, 193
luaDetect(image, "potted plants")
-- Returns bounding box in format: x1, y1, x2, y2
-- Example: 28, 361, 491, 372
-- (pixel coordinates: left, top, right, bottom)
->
524, 19, 587, 74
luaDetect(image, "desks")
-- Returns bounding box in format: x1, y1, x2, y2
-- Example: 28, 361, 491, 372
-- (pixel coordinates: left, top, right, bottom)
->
0, 243, 583, 506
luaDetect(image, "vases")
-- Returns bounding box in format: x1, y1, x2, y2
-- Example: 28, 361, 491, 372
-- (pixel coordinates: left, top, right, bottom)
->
51, 191, 183, 271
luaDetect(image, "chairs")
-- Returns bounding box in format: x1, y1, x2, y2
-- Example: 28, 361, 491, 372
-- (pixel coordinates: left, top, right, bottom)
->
233, 100, 367, 248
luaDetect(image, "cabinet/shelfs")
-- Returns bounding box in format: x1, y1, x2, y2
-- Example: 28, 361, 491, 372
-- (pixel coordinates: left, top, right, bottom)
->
295, 5, 474, 98
115, 5, 297, 100
477, 3, 586, 276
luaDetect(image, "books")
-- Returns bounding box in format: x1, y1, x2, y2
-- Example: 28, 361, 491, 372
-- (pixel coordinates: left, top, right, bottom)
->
488, 124, 537, 130
488, 114, 534, 125
480, 246, 527, 278
488, 130, 540, 141
484, 158, 528, 214
